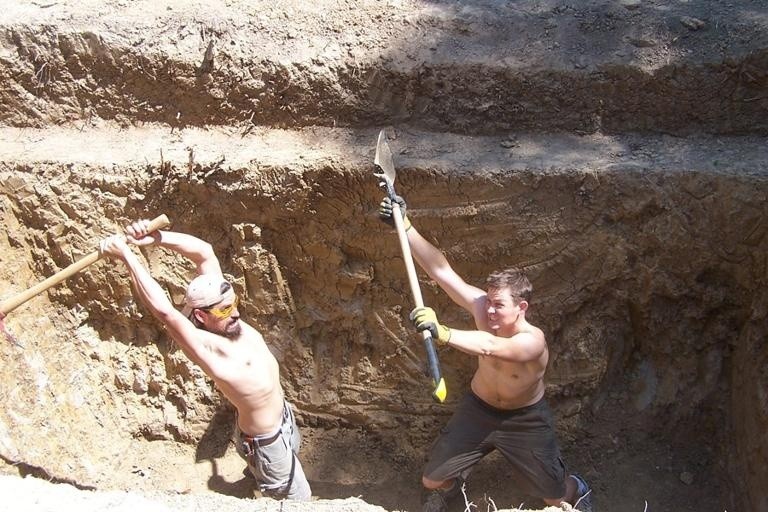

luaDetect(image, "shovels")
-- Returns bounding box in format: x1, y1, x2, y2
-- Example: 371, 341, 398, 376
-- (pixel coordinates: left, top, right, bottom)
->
374, 129, 448, 405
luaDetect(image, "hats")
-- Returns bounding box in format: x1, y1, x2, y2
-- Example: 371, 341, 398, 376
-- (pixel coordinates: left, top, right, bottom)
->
180, 274, 234, 318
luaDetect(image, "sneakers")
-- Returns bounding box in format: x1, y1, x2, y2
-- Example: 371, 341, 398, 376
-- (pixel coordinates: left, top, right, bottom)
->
421, 481, 459, 512
570, 473, 593, 512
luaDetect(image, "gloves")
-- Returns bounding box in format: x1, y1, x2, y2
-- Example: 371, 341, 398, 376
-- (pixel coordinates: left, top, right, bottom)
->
378, 194, 412, 233
409, 306, 452, 346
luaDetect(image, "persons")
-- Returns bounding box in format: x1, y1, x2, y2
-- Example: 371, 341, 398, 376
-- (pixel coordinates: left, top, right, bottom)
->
99, 219, 313, 500
378, 183, 591, 511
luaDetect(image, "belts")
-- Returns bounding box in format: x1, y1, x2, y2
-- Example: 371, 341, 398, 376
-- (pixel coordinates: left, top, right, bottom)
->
258, 408, 288, 447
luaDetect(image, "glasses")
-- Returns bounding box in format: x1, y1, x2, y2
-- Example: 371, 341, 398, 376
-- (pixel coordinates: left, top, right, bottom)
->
203, 295, 239, 321
